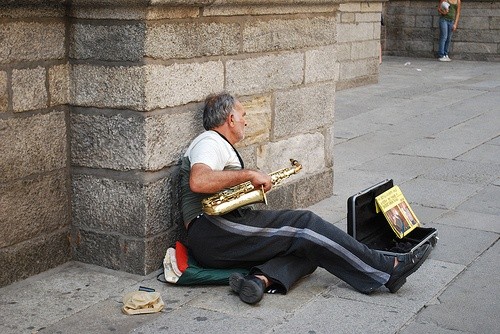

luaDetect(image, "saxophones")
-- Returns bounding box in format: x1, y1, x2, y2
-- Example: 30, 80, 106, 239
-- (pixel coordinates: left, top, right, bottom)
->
202, 158, 302, 216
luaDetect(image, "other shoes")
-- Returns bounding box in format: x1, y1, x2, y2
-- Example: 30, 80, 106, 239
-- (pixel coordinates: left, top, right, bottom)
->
439, 55, 452, 62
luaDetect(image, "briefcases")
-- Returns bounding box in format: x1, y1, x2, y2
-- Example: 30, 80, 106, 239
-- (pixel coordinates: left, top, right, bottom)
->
347, 178, 440, 265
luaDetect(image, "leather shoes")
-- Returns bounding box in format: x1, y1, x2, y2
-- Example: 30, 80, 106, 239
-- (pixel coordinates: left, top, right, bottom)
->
229, 272, 263, 304
385, 244, 432, 293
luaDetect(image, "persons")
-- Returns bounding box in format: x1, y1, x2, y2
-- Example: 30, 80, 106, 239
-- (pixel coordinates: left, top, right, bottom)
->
179, 91, 433, 306
437, 0, 461, 62
